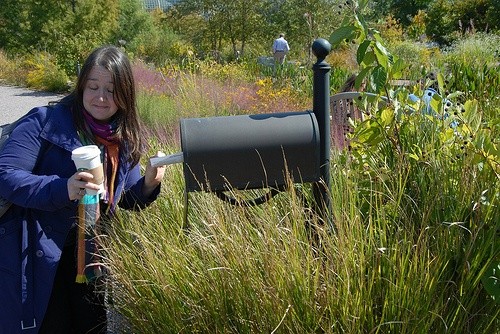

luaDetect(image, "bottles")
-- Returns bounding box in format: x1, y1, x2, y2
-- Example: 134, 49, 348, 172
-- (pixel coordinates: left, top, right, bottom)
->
80, 194, 99, 231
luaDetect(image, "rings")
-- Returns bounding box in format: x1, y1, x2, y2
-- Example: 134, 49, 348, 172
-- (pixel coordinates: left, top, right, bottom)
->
78, 189, 82, 195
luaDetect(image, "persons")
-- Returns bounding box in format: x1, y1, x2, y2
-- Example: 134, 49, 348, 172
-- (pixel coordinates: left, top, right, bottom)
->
0, 47, 167, 334
273, 33, 290, 70
334, 72, 461, 154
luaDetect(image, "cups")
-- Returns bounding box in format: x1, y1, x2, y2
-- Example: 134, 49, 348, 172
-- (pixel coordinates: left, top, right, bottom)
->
71, 145, 105, 195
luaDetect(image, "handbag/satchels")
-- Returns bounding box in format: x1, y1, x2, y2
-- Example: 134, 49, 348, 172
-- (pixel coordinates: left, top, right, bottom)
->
0, 108, 51, 221
